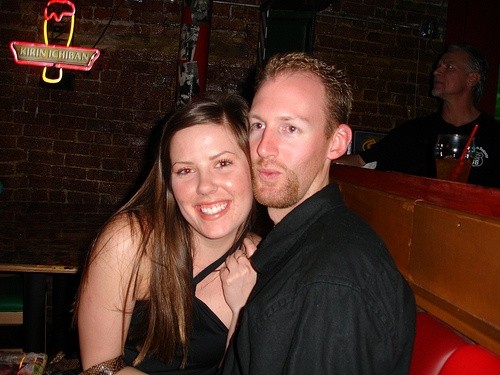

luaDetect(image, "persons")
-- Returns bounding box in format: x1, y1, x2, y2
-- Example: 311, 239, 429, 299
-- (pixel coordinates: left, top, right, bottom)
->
331, 42, 500, 190
69, 93, 263, 375
217, 51, 417, 375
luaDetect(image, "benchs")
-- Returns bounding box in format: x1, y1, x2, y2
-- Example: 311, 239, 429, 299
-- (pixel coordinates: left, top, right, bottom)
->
0, 286, 23, 324
407, 312, 500, 375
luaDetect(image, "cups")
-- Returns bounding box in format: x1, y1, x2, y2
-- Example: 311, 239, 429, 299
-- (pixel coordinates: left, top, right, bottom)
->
434, 133, 475, 183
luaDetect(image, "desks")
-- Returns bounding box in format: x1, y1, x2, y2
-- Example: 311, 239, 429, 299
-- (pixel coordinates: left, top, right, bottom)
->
0, 264, 78, 363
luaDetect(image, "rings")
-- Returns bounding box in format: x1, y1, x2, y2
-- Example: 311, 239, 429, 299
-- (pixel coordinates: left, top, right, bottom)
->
236, 254, 246, 261
